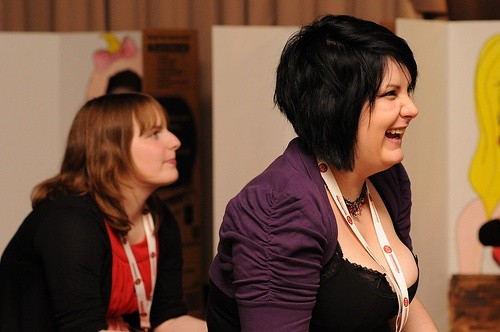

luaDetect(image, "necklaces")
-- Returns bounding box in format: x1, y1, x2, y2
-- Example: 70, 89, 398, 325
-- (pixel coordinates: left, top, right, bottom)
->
343, 177, 367, 217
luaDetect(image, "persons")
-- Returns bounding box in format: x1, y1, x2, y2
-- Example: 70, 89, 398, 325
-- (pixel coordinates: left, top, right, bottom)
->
204, 14, 440, 332
0, 68, 208, 332
